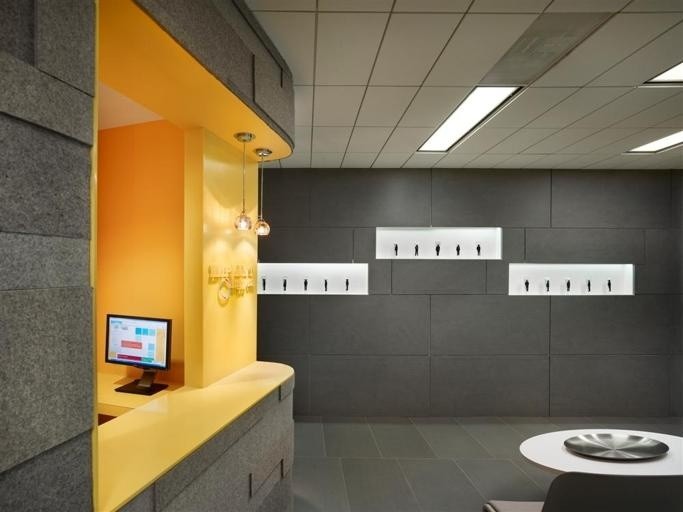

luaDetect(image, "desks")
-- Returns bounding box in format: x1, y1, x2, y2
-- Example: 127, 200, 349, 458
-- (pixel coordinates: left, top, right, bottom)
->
518, 428, 682, 478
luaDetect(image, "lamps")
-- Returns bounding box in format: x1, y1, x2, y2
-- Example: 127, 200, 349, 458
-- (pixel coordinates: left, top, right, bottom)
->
230, 130, 272, 237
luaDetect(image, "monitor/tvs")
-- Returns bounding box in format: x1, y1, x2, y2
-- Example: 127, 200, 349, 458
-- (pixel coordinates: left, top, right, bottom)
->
104, 313, 172, 396
